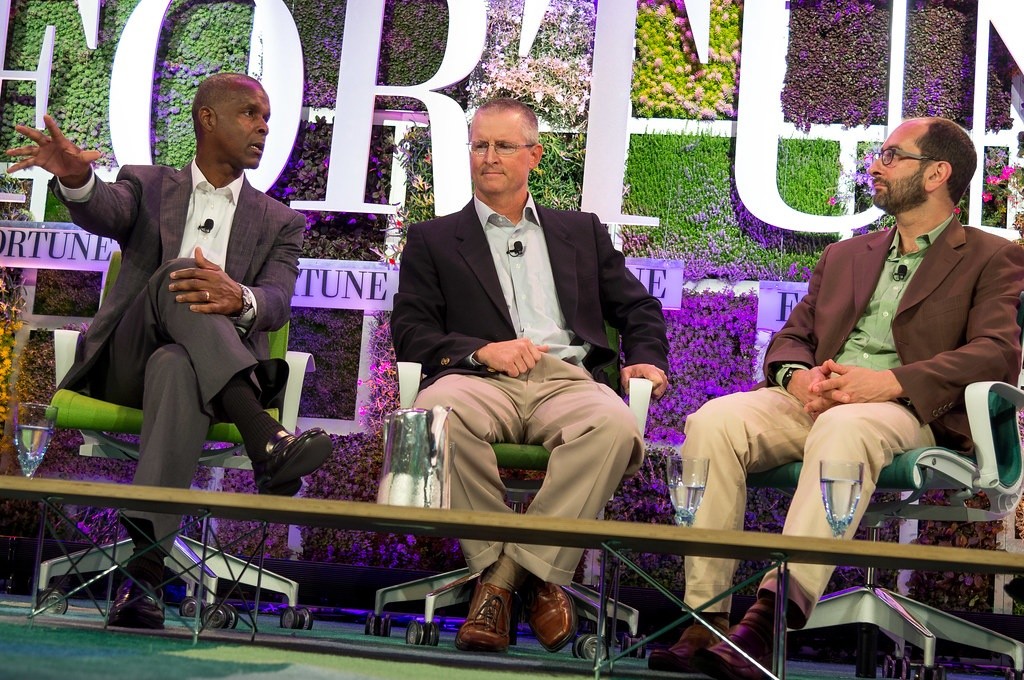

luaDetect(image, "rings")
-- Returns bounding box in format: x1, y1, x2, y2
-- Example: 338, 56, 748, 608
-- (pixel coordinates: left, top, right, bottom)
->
206, 291, 210, 301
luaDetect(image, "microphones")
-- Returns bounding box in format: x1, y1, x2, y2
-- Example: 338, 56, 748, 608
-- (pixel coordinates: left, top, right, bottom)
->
204, 219, 214, 230
514, 241, 523, 254
897, 265, 907, 279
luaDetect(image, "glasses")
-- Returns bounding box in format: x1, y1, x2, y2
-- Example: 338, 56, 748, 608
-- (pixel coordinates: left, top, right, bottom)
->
466, 140, 535, 155
872, 149, 940, 165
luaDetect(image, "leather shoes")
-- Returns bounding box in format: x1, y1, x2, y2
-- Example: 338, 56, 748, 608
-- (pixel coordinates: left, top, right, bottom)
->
693, 624, 773, 680
252, 428, 333, 495
454, 569, 513, 650
519, 573, 578, 652
108, 579, 164, 628
648, 625, 723, 671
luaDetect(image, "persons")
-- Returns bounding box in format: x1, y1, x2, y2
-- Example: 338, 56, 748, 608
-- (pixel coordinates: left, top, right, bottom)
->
644, 117, 1023, 680
7, 73, 333, 630
390, 100, 669, 655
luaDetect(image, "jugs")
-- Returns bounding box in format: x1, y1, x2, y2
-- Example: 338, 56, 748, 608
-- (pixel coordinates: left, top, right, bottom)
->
377, 405, 450, 509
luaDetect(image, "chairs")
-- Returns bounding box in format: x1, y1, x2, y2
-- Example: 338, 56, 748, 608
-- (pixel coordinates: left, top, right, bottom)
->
370, 317, 652, 661
36, 249, 316, 626
777, 292, 1024, 679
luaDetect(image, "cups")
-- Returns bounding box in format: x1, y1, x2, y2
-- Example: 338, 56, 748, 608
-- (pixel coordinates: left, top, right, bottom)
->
667, 456, 710, 528
13, 402, 59, 477
449, 442, 455, 474
819, 460, 865, 537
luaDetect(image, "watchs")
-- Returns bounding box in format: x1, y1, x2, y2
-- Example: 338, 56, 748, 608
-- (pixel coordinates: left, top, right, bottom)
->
230, 284, 251, 320
783, 369, 798, 389
